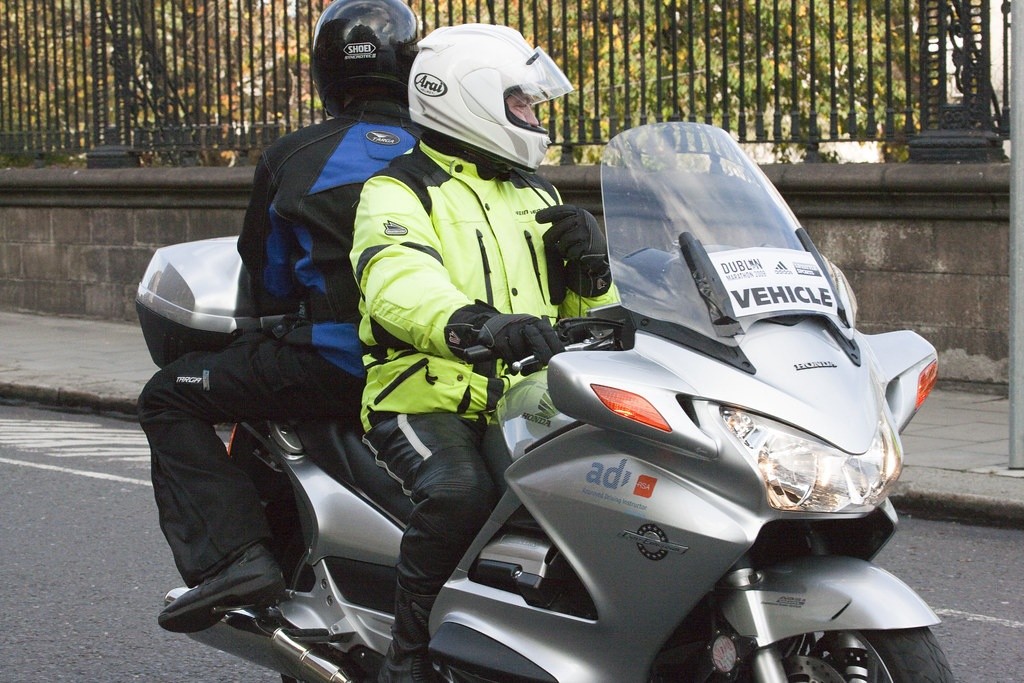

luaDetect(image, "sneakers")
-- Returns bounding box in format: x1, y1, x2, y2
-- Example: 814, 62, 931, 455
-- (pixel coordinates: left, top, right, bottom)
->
158, 544, 286, 633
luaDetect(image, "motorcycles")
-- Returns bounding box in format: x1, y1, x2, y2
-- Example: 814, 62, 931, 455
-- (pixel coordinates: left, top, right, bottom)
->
140, 140, 951, 682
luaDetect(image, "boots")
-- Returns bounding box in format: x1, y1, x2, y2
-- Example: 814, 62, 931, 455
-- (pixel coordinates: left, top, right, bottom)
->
377, 576, 439, 683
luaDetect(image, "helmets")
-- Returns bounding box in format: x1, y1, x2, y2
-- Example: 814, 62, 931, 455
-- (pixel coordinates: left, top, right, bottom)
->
312, 0, 419, 116
408, 23, 575, 172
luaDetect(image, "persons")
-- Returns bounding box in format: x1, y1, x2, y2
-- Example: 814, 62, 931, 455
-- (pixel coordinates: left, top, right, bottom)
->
211, 112, 297, 165
348, 23, 627, 683
136, 0, 422, 632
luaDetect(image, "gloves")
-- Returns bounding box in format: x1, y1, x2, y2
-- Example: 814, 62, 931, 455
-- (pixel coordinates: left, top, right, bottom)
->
536, 205, 612, 305
444, 299, 566, 376
